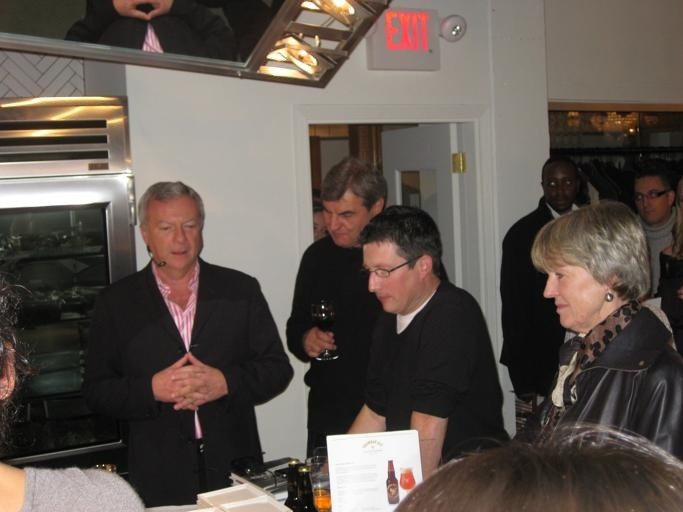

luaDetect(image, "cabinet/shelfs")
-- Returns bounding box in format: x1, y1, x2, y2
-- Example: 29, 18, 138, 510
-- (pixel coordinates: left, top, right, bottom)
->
549, 102, 683, 197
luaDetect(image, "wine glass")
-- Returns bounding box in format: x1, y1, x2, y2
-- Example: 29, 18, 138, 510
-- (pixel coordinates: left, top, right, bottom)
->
310, 297, 340, 361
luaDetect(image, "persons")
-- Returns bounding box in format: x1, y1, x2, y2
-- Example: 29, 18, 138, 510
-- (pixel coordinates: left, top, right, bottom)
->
393, 428, 683, 512
82, 182, 293, 507
288, 156, 449, 458
0, 274, 145, 512
500, 156, 683, 403
319, 206, 511, 483
513, 200, 683, 462
313, 188, 330, 241
65, 0, 237, 62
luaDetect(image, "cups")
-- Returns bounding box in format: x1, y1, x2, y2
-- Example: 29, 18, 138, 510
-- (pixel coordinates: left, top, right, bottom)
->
305, 455, 331, 511
400, 467, 415, 489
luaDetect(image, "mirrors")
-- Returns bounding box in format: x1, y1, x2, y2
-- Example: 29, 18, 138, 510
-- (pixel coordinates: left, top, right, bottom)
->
1, 0, 290, 76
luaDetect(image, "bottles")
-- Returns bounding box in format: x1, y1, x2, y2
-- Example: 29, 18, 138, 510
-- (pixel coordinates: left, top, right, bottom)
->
385, 459, 400, 504
295, 465, 318, 512
284, 458, 301, 511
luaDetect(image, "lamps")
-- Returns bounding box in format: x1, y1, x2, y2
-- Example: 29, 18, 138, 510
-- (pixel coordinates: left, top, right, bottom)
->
439, 10, 467, 43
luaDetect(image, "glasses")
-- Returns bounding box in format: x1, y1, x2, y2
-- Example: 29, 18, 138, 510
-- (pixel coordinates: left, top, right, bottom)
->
365, 255, 423, 279
635, 190, 667, 200
